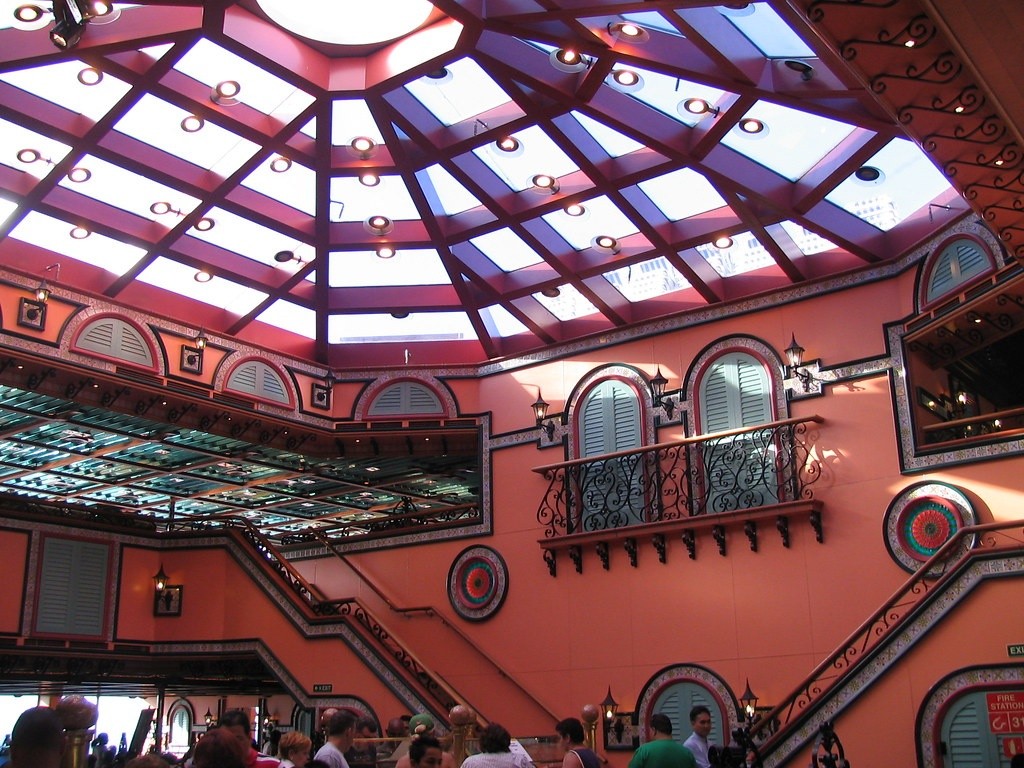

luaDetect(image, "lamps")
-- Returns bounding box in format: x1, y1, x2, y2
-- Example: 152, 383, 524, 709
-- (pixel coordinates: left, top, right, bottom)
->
649, 367, 676, 419
741, 678, 764, 739
785, 332, 813, 381
317, 368, 337, 400
188, 328, 208, 364
153, 563, 174, 611
601, 686, 624, 742
531, 390, 555, 438
28, 277, 52, 319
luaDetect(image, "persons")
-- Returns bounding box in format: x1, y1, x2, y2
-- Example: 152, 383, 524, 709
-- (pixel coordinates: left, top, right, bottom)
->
1010, 753, 1024, 768
0, 706, 713, 768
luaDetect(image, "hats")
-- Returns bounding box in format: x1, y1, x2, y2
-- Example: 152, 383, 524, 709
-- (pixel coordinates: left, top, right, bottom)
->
408, 713, 435, 736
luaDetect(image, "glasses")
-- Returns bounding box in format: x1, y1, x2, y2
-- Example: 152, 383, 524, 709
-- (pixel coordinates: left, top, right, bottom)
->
360, 730, 377, 739
